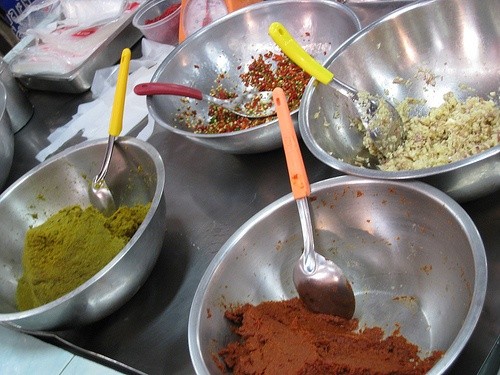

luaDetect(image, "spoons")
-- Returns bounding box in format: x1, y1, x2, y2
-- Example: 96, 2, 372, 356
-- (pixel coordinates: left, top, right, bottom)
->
270, 22, 403, 154
133, 83, 277, 117
87, 49, 130, 214
271, 88, 354, 321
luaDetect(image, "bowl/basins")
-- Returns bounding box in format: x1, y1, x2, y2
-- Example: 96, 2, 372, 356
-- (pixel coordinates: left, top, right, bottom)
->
187, 175, 487, 375
132, 0, 182, 47
146, 0, 362, 154
0, 82, 15, 193
0, 136, 165, 330
298, 0, 500, 201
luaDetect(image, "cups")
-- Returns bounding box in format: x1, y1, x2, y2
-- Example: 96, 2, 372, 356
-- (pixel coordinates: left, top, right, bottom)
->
0, 60, 35, 134
